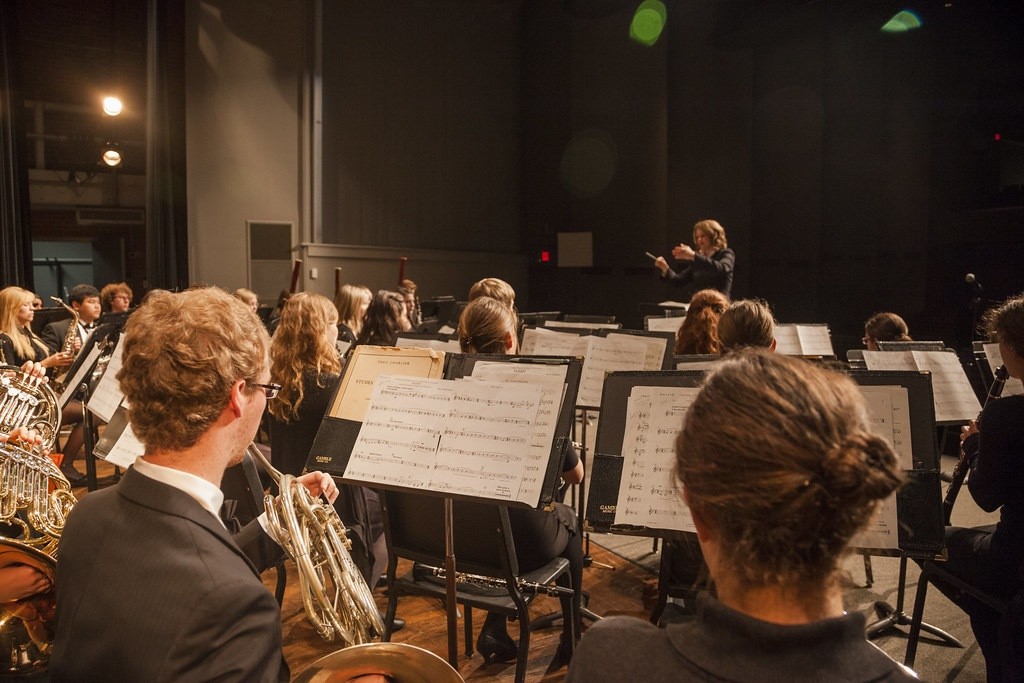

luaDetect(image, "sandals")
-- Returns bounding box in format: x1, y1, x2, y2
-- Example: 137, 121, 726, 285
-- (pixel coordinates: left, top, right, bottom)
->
58, 463, 87, 486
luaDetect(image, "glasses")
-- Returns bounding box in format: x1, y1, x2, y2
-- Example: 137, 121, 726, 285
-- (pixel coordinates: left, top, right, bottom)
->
248, 383, 281, 399
249, 302, 260, 307
862, 337, 877, 345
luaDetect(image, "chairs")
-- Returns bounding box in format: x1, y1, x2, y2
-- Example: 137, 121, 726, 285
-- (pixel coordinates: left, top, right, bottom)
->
217, 378, 1024, 683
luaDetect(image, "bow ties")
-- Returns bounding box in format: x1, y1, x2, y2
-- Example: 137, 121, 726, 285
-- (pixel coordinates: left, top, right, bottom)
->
79, 321, 95, 333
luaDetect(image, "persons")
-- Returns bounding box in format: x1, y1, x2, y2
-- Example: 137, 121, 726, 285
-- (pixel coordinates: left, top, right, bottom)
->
715, 298, 777, 354
268, 290, 406, 637
675, 289, 730, 355
468, 277, 520, 356
0, 286, 102, 486
41, 283, 309, 441
45, 293, 339, 683
915, 298, 1024, 683
860, 312, 913, 350
457, 296, 583, 666
657, 219, 736, 309
565, 349, 922, 683
330, 280, 427, 349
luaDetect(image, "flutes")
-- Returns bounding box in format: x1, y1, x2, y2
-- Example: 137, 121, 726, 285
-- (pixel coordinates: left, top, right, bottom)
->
942, 362, 1009, 527
412, 561, 576, 598
415, 296, 423, 326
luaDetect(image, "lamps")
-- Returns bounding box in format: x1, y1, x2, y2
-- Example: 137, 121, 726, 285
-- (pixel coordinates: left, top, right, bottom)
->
97, 141, 127, 170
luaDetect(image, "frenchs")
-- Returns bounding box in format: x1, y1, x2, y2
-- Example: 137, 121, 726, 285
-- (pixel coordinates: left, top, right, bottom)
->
248, 439, 464, 683
1, 338, 83, 678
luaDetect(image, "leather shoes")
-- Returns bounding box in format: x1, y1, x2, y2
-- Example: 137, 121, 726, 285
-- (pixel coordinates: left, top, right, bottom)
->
382, 615, 405, 630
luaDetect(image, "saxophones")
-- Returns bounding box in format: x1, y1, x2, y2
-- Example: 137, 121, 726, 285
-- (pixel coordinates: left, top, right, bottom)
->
48, 295, 82, 396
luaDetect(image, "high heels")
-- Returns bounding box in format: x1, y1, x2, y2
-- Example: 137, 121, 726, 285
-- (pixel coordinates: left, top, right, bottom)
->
476, 633, 519, 663
560, 632, 580, 663
